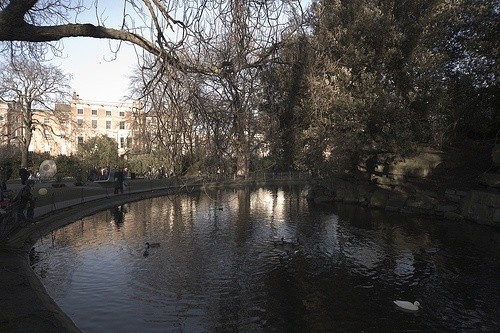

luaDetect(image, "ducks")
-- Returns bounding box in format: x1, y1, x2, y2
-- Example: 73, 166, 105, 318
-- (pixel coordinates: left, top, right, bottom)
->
144, 242, 162, 249
273, 237, 302, 248
393, 299, 423, 312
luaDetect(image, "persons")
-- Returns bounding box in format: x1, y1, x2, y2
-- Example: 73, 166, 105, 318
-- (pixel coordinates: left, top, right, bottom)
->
15, 180, 36, 219
113, 168, 124, 194
19, 165, 30, 185
0, 166, 10, 191
